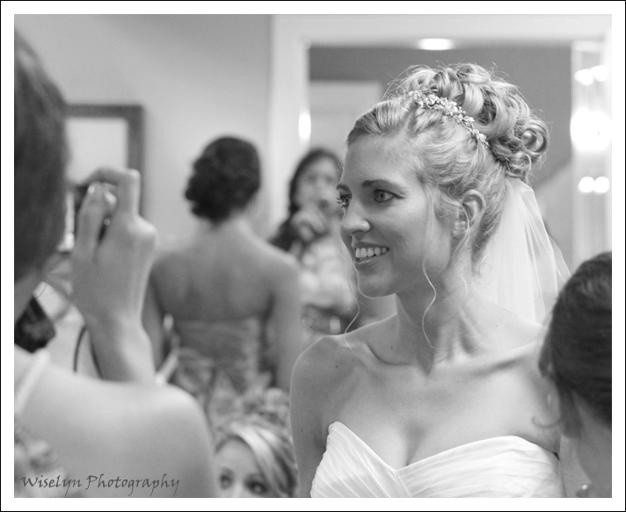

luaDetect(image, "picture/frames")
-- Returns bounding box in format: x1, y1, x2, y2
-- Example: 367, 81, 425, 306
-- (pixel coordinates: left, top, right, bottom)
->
57, 104, 143, 254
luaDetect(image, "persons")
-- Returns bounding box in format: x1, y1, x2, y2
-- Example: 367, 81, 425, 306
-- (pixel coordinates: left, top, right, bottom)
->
211, 415, 298, 497
288, 59, 577, 498
530, 250, 611, 497
140, 136, 305, 436
14, 29, 220, 496
266, 148, 397, 337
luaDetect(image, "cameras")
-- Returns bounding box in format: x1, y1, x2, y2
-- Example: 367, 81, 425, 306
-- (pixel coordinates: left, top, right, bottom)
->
80, 182, 119, 215
